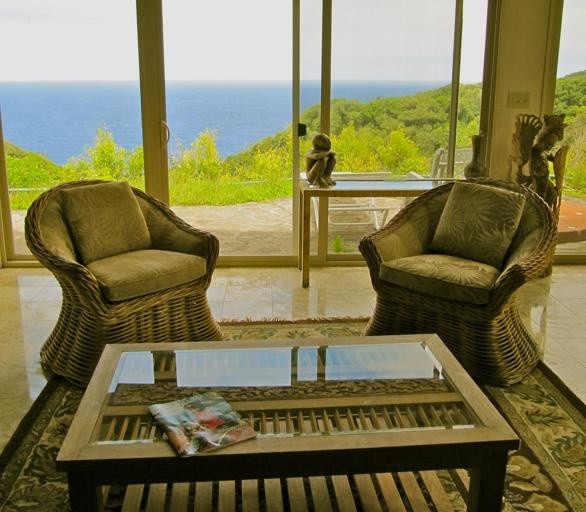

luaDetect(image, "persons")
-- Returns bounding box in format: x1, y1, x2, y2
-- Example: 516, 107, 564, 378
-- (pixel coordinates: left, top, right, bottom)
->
305, 132, 338, 189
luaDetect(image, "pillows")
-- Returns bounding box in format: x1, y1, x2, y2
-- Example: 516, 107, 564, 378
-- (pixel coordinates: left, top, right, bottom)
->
430, 182, 526, 269
59, 180, 152, 264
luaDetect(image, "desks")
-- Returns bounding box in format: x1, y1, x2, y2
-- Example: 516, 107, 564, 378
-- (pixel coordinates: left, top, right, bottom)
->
299, 178, 464, 288
55, 328, 520, 508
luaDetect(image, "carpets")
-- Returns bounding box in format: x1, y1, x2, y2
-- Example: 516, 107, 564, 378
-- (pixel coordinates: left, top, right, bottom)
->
0, 316, 585, 511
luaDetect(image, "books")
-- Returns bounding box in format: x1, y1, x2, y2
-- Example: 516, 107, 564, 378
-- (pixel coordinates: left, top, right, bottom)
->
147, 389, 258, 459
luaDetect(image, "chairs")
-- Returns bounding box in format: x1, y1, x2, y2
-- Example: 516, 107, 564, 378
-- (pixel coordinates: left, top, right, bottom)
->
359, 176, 559, 387
299, 139, 531, 269
24, 178, 225, 388
